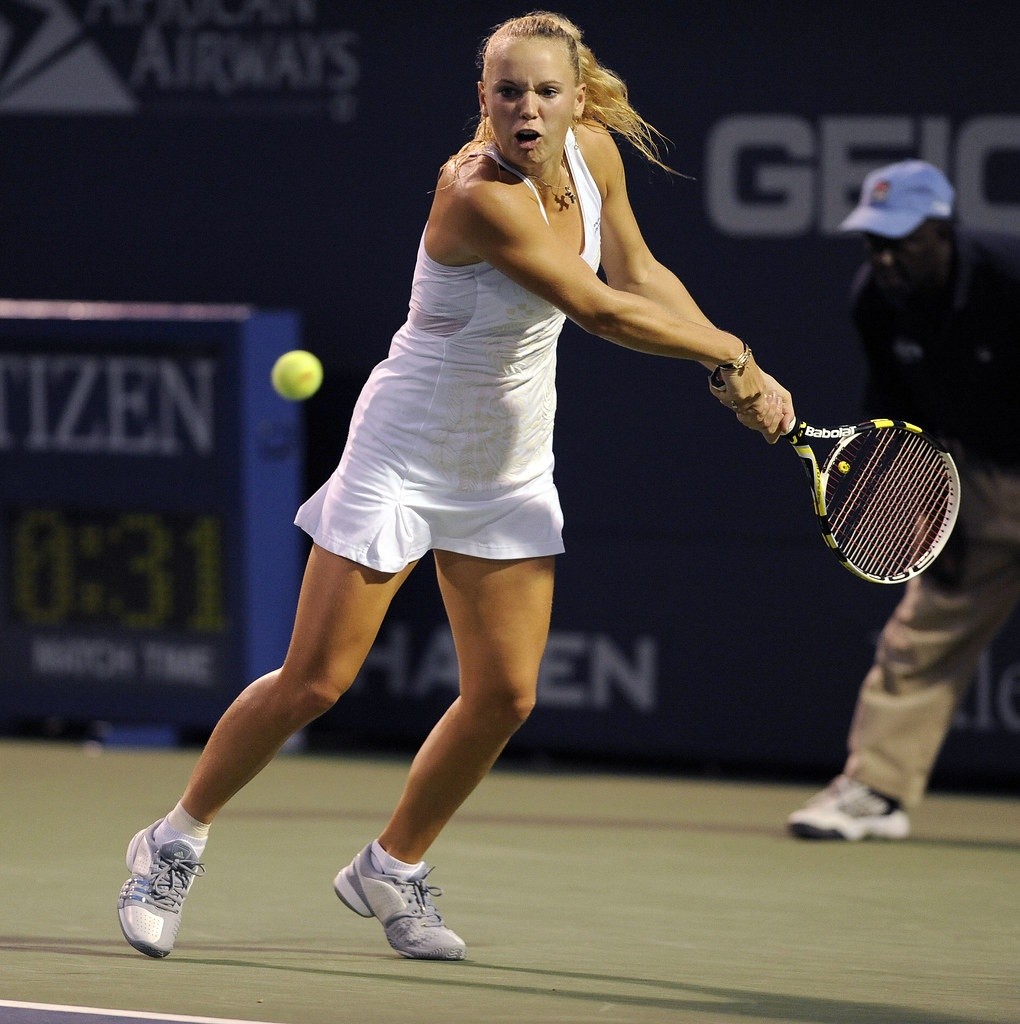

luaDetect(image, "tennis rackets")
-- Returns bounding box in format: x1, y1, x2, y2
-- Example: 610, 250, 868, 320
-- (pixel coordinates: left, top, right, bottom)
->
709, 363, 962, 586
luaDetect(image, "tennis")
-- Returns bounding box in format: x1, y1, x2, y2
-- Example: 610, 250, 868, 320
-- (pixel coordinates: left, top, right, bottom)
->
272, 350, 322, 400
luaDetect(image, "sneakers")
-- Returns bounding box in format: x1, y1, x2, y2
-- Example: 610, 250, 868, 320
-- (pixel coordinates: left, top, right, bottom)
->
333, 841, 466, 963
786, 776, 911, 843
116, 815, 208, 959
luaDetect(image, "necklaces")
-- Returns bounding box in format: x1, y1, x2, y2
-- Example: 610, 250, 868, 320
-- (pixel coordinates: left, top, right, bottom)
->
521, 152, 578, 205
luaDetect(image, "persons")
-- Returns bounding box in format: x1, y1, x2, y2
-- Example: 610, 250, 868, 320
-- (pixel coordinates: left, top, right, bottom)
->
110, 13, 793, 960
781, 159, 1018, 842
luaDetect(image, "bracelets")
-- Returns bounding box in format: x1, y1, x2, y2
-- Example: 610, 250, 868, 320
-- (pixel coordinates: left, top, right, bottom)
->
718, 338, 752, 370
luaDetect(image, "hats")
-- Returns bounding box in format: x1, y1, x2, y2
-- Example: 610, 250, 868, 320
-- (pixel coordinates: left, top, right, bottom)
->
840, 158, 956, 238
